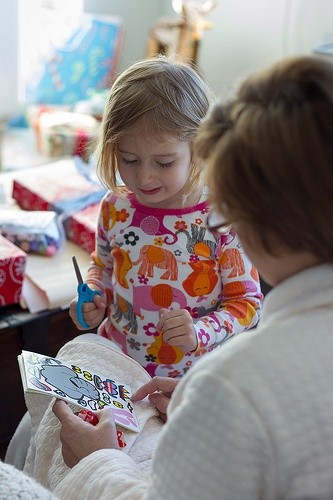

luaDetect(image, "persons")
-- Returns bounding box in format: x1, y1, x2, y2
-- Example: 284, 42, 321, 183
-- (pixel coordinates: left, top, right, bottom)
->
63, 52, 263, 381
0, 50, 333, 500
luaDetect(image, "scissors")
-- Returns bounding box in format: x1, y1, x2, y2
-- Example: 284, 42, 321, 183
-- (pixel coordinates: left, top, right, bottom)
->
72, 256, 105, 329
